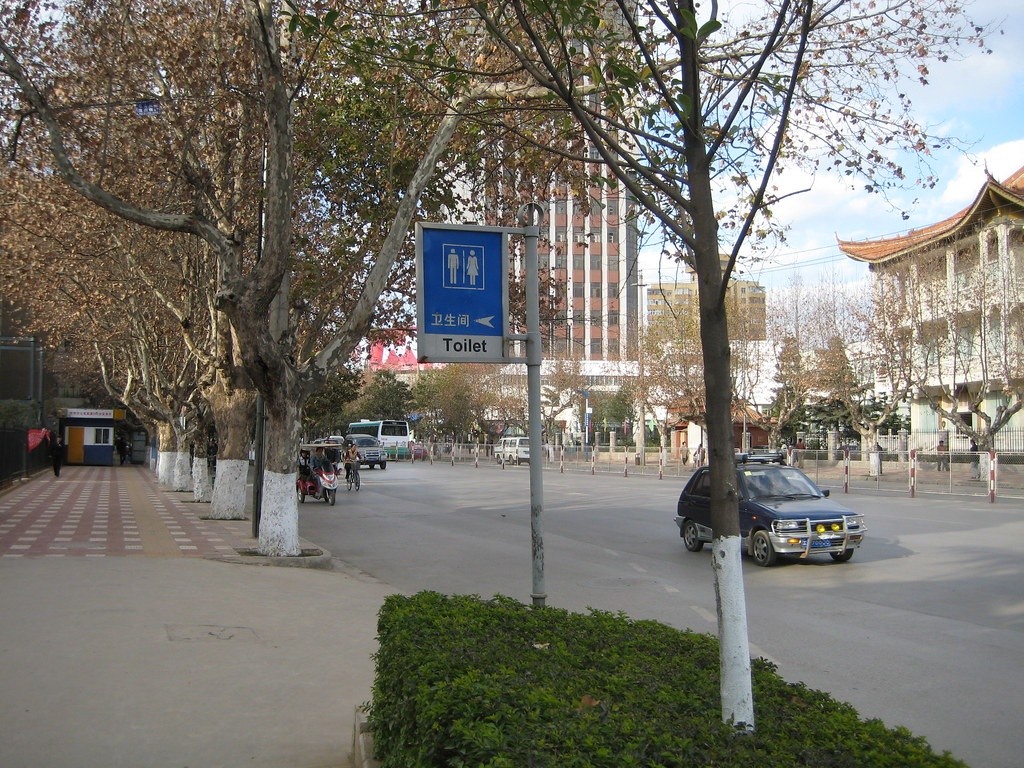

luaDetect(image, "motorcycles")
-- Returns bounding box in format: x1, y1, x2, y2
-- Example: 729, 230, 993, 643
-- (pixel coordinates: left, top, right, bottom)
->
296, 443, 344, 505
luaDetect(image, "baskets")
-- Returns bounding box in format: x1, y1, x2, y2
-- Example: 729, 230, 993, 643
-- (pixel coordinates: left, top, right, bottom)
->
350, 462, 361, 470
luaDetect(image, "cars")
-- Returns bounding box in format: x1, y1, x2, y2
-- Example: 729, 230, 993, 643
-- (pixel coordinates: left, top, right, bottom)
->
673, 452, 866, 565
413, 444, 429, 460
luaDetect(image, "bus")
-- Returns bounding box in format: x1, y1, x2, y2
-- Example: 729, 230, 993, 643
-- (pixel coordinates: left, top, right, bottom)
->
349, 419, 410, 459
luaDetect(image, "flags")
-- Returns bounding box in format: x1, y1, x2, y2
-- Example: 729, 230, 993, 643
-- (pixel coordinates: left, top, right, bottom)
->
649, 416, 654, 432
603, 418, 607, 432
624, 422, 627, 435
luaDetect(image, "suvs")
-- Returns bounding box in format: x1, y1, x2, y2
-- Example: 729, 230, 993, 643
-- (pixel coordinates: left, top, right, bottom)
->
346, 434, 387, 470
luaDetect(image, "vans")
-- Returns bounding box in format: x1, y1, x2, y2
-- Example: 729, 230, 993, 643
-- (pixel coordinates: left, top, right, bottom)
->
494, 437, 530, 465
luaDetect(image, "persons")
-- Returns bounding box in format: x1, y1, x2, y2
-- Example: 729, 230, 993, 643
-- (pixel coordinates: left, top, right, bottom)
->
780, 438, 807, 469
679, 441, 706, 466
47, 435, 67, 478
936, 440, 950, 471
298, 441, 342, 482
114, 437, 127, 465
968, 438, 981, 479
344, 444, 363, 482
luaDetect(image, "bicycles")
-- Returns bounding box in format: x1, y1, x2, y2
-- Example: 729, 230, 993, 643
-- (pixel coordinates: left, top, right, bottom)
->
345, 459, 363, 491
207, 455, 216, 472
